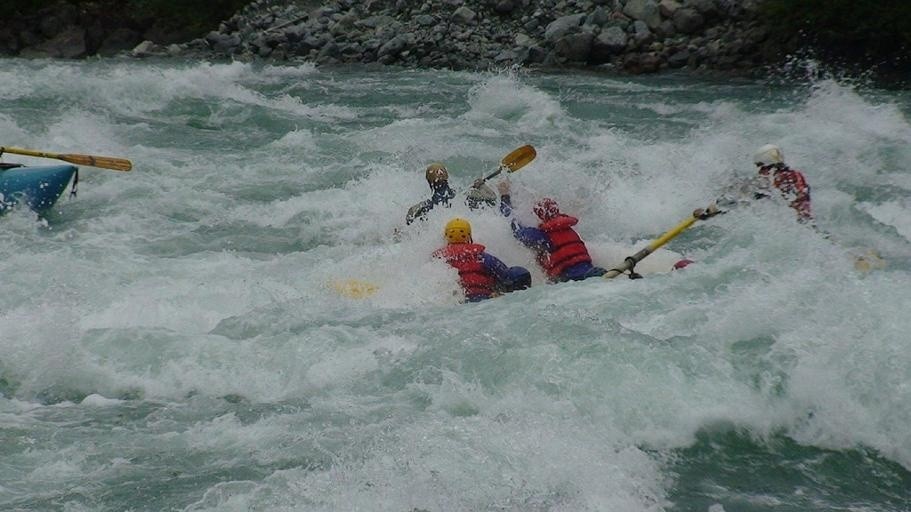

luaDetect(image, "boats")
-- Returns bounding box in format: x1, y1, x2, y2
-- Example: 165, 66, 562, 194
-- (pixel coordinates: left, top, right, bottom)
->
316, 232, 888, 297
0, 162, 79, 228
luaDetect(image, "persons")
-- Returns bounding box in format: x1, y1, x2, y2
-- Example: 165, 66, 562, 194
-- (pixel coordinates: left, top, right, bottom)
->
695, 142, 812, 227
405, 164, 496, 225
432, 216, 532, 305
496, 177, 595, 283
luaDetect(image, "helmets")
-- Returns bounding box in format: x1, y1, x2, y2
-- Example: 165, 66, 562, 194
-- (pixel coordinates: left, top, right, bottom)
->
533, 197, 560, 220
426, 162, 449, 182
445, 218, 472, 243
755, 144, 784, 168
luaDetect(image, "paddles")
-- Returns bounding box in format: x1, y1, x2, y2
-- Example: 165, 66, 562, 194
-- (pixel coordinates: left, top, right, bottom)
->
484, 144, 536, 180
3, 147, 132, 173
